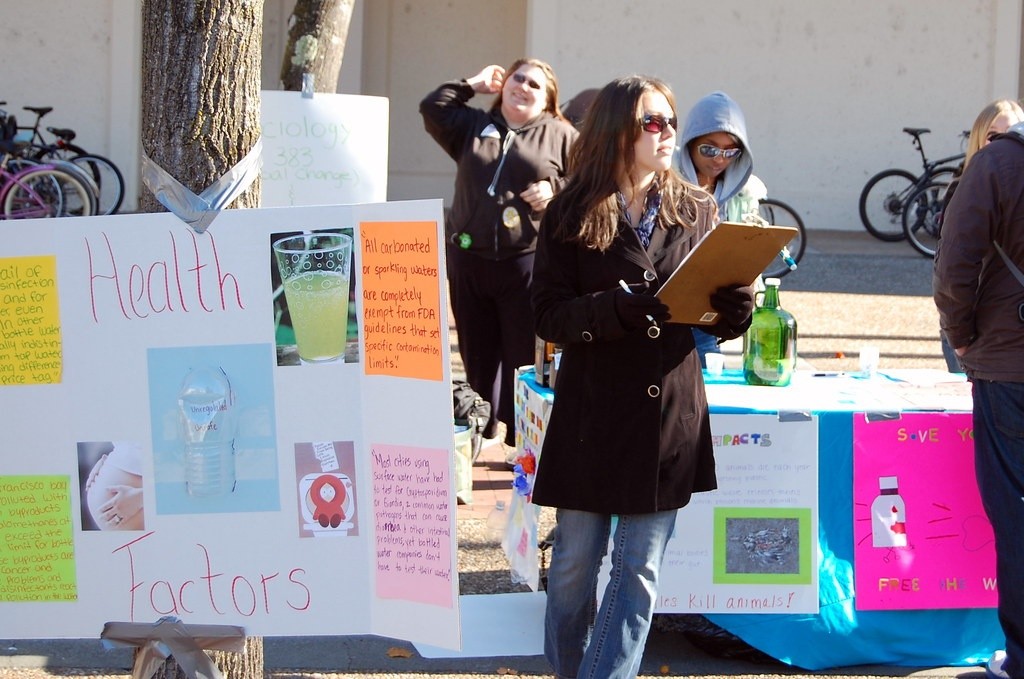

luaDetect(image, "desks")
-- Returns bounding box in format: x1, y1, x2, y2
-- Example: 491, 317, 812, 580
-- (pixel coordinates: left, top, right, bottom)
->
514, 365, 1006, 669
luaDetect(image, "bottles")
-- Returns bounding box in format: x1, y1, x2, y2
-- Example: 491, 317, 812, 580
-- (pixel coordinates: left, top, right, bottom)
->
744, 278, 798, 385
177, 361, 236, 496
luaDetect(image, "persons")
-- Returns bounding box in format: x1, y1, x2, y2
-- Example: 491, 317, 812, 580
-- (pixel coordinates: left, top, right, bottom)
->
934, 124, 1024, 679
671, 90, 767, 368
939, 98, 1023, 373
524, 75, 755, 679
418, 59, 580, 465
86, 441, 145, 531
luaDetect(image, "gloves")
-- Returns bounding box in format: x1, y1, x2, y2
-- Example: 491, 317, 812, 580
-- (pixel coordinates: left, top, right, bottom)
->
616, 280, 672, 328
710, 284, 754, 329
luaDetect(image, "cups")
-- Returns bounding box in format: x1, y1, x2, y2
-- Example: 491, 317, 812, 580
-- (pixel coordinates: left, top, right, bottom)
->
706, 353, 724, 375
272, 233, 352, 365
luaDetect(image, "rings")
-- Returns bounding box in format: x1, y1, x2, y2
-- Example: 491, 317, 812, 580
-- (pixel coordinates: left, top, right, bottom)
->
114, 516, 121, 522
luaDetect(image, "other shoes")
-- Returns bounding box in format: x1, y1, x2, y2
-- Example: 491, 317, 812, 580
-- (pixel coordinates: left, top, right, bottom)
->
482, 435, 500, 449
504, 449, 519, 470
986, 649, 1011, 678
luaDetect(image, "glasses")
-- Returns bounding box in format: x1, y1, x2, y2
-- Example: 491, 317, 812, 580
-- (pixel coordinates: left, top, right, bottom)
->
639, 116, 678, 132
512, 72, 546, 96
693, 141, 742, 161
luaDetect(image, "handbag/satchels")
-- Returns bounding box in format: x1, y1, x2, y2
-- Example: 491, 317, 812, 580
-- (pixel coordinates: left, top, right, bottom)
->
452, 380, 491, 506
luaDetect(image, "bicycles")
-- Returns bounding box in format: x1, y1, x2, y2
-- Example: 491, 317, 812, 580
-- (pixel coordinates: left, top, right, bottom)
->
755, 199, 806, 282
0, 99, 125, 219
855, 127, 979, 257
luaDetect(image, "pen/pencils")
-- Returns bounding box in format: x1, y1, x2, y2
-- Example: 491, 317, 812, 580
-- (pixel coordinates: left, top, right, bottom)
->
778, 249, 797, 270
618, 279, 658, 327
